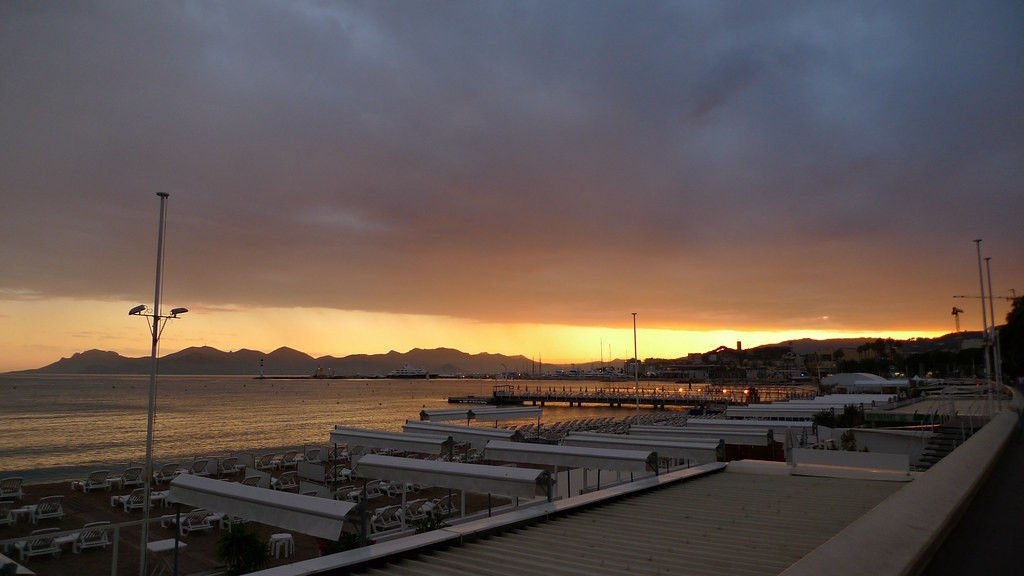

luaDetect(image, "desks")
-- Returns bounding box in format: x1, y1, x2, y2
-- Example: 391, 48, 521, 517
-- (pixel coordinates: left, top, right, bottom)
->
267, 533, 295, 559
9, 508, 32, 524
105, 479, 123, 492
146, 538, 187, 575
54, 535, 78, 555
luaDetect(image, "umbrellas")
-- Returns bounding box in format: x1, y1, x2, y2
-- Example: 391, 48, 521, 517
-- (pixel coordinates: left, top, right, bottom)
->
783, 426, 800, 458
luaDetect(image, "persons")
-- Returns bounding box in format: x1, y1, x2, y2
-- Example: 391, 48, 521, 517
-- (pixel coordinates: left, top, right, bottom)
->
689, 381, 693, 391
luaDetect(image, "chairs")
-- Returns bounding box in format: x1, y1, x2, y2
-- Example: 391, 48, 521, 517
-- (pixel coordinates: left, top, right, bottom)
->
0, 443, 486, 575
485, 412, 727, 435
